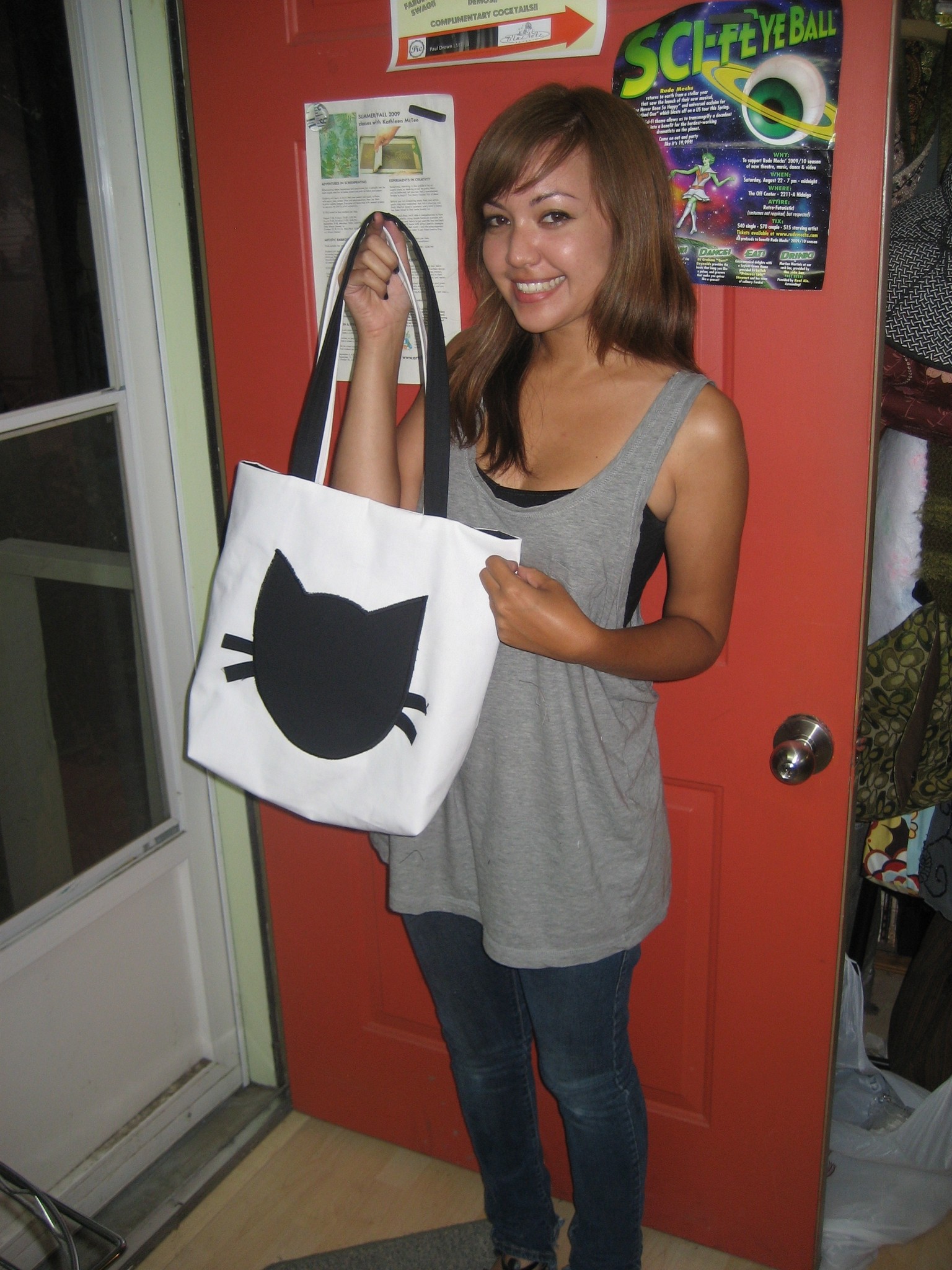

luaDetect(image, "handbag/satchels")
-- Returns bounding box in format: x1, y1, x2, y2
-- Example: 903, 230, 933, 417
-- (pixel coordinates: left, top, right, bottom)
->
789, 951, 952, 1270
185, 205, 532, 836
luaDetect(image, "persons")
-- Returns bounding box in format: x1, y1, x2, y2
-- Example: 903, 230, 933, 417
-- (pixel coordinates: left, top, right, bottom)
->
327, 81, 750, 1270
374, 126, 401, 153
